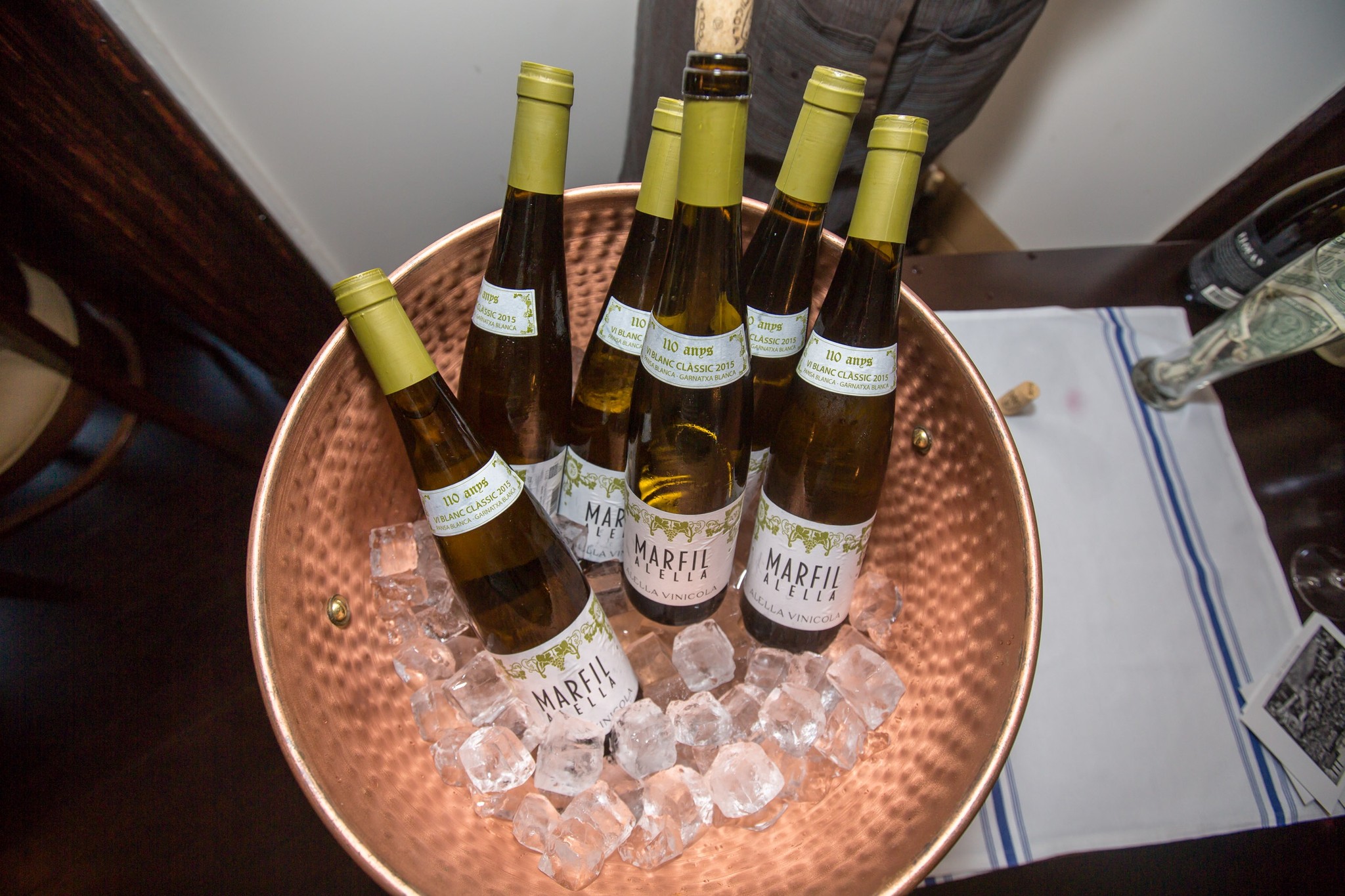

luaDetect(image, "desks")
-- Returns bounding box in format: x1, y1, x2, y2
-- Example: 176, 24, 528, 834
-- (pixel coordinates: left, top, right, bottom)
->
187, 244, 1345, 896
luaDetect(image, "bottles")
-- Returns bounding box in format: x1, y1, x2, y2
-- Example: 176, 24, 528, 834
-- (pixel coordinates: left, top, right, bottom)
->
1176, 164, 1345, 318
331, 264, 646, 752
619, 50, 759, 625
739, 113, 931, 653
742, 65, 866, 499
554, 95, 685, 570
457, 64, 575, 518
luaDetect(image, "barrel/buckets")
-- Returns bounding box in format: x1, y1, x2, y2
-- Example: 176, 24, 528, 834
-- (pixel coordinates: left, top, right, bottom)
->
244, 177, 1043, 893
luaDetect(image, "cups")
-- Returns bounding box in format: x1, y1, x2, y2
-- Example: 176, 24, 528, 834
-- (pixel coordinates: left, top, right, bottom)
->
1129, 236, 1344, 410
